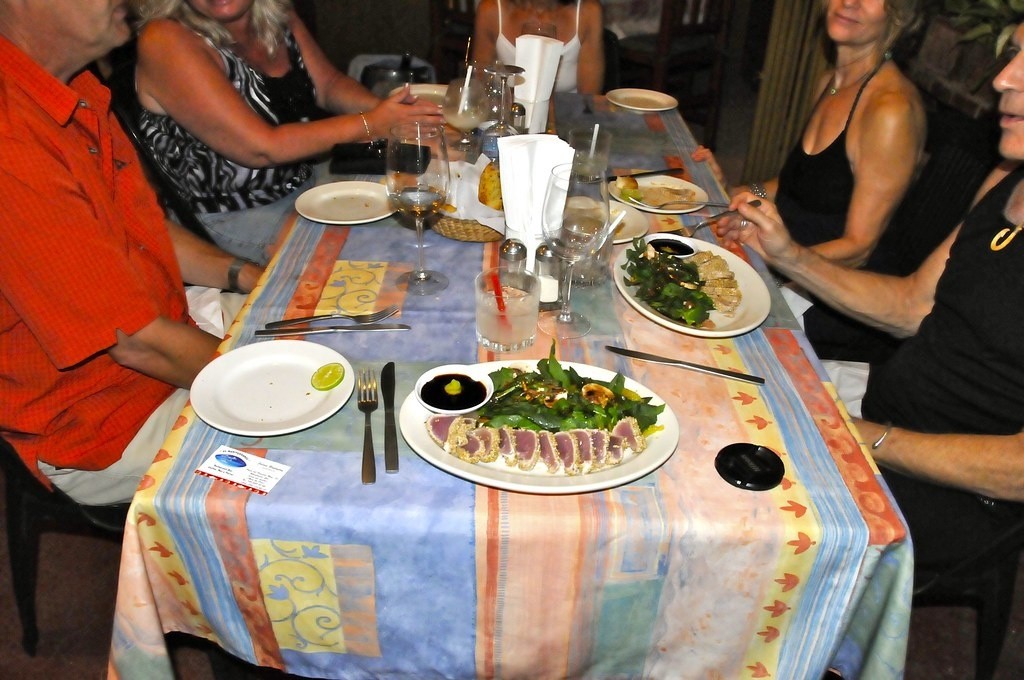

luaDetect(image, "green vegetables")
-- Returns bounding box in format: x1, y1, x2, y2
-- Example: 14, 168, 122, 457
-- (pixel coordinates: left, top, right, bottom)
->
621, 238, 716, 328
473, 338, 667, 437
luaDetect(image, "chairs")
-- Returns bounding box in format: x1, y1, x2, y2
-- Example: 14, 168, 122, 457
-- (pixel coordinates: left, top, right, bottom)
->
880, 100, 995, 277
428, 0, 476, 66
93, 56, 214, 245
609, 0, 732, 152
907, 495, 1024, 678
602, 28, 619, 95
0, 434, 129, 655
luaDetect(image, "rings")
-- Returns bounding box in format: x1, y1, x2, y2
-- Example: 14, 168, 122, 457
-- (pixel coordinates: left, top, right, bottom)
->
741, 219, 747, 229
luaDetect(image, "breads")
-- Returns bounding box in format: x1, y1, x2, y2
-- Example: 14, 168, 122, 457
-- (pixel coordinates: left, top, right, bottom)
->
683, 252, 741, 316
616, 176, 638, 191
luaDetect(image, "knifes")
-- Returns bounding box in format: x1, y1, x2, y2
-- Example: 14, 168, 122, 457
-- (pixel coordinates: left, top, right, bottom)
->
605, 346, 765, 385
254, 323, 411, 336
379, 362, 399, 472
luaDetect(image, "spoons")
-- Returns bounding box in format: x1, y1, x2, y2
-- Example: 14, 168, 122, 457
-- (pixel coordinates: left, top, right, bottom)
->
628, 196, 730, 209
566, 196, 597, 210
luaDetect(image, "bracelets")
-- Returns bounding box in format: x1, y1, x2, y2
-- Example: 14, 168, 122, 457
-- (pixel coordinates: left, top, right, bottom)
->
872, 420, 891, 448
359, 111, 373, 145
228, 256, 249, 293
750, 184, 767, 199
773, 273, 784, 287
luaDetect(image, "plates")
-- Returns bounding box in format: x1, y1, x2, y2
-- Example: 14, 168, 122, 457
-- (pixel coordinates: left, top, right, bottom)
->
398, 357, 681, 497
608, 169, 708, 213
563, 200, 649, 243
605, 87, 773, 339
183, 178, 400, 439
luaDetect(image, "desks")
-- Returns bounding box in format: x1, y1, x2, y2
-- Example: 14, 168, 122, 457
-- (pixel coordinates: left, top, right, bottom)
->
109, 91, 916, 680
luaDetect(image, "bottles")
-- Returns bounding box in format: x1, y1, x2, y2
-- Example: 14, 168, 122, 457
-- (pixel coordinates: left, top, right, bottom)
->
510, 101, 525, 135
498, 238, 562, 311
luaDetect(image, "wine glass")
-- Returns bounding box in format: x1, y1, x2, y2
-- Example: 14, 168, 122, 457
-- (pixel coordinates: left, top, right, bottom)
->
537, 162, 610, 339
442, 78, 489, 152
483, 65, 525, 165
386, 122, 450, 296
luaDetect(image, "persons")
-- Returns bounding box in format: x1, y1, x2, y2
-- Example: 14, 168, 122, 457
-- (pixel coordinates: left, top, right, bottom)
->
472, 0, 605, 129
0, 0, 266, 506
132, 0, 466, 273
690, 0, 929, 299
716, 17, 1024, 595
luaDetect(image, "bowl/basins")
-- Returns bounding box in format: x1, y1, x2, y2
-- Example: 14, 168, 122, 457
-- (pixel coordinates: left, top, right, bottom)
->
415, 364, 494, 415
479, 120, 518, 163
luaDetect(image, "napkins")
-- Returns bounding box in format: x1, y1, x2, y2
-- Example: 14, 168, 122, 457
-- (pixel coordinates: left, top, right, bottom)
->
496, 34, 575, 298
821, 358, 870, 419
780, 286, 814, 335
183, 282, 223, 339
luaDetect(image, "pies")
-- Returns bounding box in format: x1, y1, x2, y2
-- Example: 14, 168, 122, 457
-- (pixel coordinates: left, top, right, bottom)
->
478, 162, 503, 211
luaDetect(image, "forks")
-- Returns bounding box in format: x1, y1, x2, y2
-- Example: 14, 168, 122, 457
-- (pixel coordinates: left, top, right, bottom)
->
265, 304, 399, 328
357, 367, 378, 485
656, 200, 762, 237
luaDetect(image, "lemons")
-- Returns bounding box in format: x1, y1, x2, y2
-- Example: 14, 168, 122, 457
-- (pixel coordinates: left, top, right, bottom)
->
311, 363, 345, 390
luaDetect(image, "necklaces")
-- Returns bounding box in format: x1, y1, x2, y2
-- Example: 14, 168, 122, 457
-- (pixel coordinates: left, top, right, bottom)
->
532, 0, 549, 30
829, 51, 882, 95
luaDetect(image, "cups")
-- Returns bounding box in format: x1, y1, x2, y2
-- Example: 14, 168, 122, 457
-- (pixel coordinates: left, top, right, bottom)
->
475, 266, 540, 353
511, 24, 556, 135
569, 125, 611, 184
